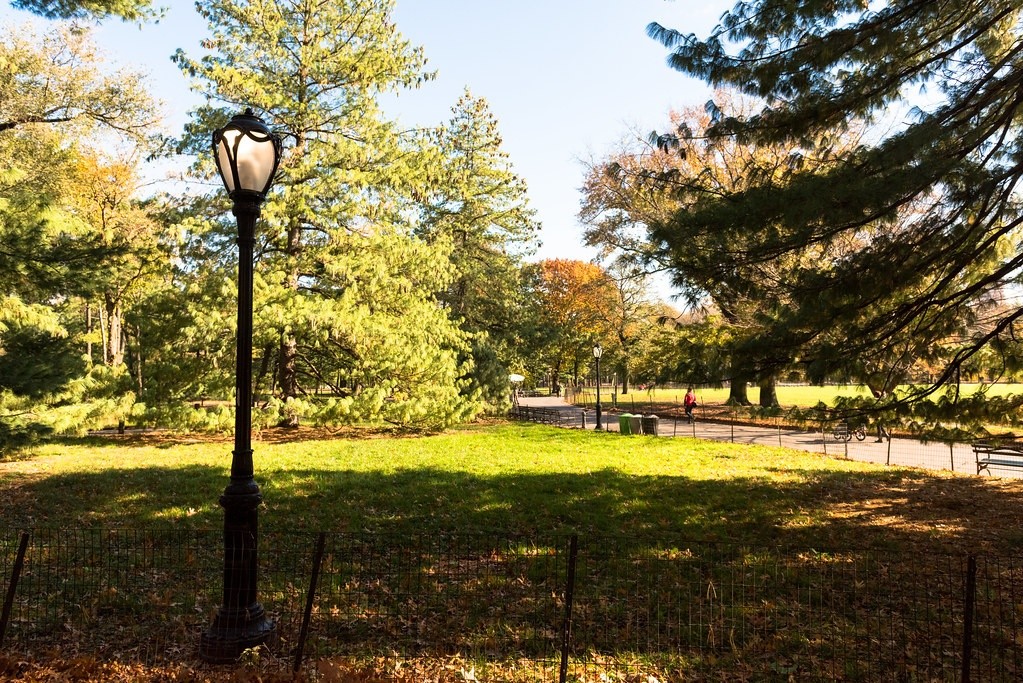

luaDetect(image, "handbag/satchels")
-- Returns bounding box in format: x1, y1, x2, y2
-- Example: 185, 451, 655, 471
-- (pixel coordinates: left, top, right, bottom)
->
692, 402, 698, 408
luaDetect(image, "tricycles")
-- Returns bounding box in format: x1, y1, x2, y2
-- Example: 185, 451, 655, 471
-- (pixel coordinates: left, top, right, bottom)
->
833, 422, 866, 441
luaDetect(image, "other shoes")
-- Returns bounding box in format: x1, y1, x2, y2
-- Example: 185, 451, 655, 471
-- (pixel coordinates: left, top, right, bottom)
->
692, 417, 695, 422
688, 421, 691, 423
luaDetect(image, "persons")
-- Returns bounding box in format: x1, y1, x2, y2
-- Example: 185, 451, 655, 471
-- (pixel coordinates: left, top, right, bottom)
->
684, 386, 696, 423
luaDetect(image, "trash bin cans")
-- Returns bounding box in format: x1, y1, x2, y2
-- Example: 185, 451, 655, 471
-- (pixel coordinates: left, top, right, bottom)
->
618, 412, 659, 435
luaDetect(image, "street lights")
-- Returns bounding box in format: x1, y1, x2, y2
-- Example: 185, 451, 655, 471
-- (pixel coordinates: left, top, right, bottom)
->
591, 342, 607, 432
203, 108, 287, 667
